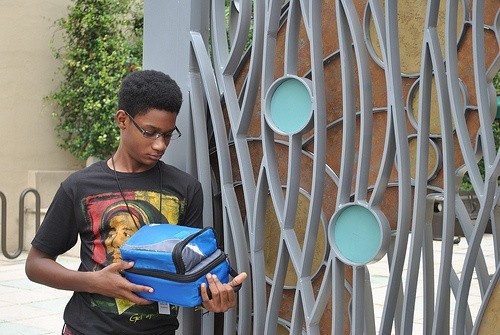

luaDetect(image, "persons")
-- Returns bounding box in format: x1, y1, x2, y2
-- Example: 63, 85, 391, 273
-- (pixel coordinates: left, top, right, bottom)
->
26, 69, 247, 335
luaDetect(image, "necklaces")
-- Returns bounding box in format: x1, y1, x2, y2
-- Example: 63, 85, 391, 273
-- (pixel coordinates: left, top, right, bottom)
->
112, 154, 162, 231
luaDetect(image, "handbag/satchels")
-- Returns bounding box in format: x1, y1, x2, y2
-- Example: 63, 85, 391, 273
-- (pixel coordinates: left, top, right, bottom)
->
120, 223, 242, 308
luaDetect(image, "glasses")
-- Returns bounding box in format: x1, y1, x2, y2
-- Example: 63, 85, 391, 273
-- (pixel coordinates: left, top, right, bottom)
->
125, 112, 182, 140
